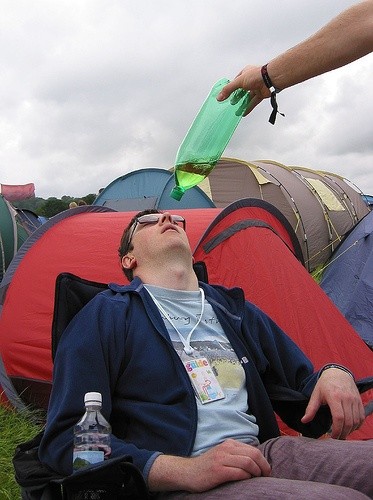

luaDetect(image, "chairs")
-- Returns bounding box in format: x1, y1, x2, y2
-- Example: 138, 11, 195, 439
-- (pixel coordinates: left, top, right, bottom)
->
13, 273, 373, 500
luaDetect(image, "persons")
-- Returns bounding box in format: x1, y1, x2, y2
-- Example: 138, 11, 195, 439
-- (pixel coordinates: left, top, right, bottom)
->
216, 0, 373, 118
36, 210, 373, 500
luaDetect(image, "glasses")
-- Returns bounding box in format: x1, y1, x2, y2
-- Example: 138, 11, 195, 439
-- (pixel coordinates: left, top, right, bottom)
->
125, 213, 185, 255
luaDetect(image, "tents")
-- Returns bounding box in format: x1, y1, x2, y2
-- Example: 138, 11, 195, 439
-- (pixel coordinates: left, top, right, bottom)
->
0, 157, 373, 443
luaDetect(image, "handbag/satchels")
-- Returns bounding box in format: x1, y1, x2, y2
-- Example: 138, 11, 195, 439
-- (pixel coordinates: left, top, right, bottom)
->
12, 430, 160, 500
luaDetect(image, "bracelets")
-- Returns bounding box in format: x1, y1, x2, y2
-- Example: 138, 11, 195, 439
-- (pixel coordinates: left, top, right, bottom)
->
261, 64, 285, 124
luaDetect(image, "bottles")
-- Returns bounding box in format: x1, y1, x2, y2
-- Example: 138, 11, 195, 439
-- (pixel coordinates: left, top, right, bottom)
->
169, 78, 251, 202
73, 392, 112, 474
